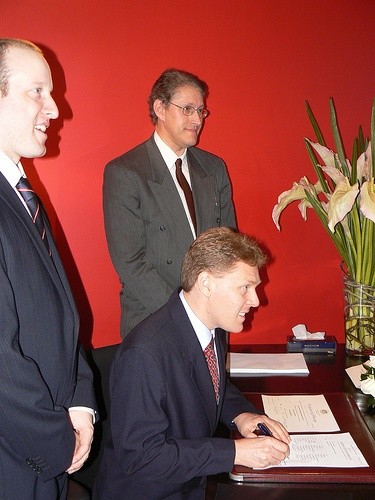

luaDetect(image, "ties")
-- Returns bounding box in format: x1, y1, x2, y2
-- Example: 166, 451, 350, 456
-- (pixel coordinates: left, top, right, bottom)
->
15, 178, 62, 312
203, 332, 222, 409
172, 156, 199, 239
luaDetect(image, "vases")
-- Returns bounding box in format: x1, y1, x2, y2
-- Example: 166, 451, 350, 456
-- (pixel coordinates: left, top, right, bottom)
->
344, 274, 375, 355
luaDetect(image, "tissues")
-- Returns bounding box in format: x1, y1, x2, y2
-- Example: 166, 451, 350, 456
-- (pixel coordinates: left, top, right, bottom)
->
288, 324, 338, 353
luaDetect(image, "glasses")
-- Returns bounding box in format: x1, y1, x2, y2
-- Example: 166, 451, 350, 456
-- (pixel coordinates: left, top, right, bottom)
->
163, 99, 211, 117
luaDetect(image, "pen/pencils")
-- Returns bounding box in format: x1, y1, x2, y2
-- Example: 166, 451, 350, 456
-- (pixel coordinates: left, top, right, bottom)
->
257, 423, 290, 459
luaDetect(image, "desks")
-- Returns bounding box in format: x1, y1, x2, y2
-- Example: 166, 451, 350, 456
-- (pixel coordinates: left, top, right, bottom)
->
206, 343, 374, 499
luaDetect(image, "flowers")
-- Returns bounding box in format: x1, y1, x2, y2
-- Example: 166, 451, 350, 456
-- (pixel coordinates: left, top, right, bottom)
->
272, 96, 375, 275
345, 355, 375, 415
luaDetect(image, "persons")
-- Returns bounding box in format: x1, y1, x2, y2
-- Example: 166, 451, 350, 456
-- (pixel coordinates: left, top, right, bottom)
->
0, 38, 103, 500
92, 226, 292, 500
100, 67, 240, 340
